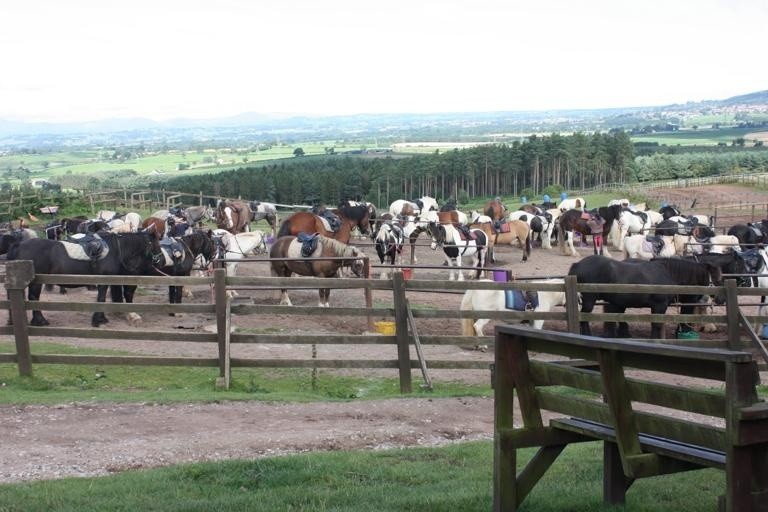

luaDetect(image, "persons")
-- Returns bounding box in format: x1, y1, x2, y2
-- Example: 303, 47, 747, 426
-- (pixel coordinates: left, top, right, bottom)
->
163, 216, 190, 237
586, 212, 607, 255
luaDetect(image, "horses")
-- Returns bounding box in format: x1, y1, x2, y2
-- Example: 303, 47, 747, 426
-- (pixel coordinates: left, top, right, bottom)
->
0, 195, 768, 352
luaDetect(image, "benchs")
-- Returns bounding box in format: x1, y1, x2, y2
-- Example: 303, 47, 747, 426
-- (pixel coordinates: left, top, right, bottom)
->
487, 321, 768, 511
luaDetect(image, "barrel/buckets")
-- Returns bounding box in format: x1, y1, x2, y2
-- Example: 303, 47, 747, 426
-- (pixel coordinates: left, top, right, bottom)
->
373, 320, 395, 335
401, 267, 412, 280
543, 194, 550, 203
560, 193, 568, 201
678, 330, 700, 339
493, 270, 513, 281
